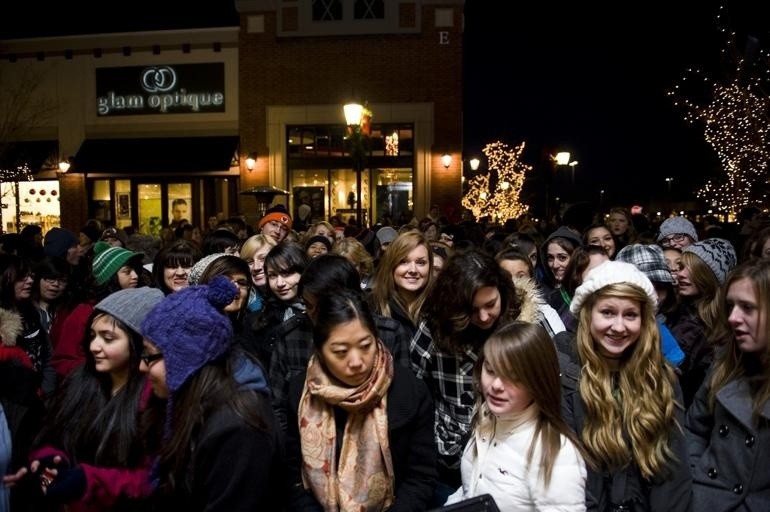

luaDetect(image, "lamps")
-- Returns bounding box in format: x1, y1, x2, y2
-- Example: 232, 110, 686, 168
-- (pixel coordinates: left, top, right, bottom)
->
244, 151, 257, 171
57, 152, 73, 174
384, 125, 400, 157
439, 145, 452, 167
465, 154, 481, 172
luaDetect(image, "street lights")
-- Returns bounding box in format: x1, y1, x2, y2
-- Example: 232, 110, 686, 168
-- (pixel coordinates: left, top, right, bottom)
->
343, 102, 367, 235
552, 151, 579, 199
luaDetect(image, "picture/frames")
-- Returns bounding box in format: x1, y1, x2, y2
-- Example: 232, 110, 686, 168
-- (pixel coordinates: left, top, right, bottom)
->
115, 192, 131, 220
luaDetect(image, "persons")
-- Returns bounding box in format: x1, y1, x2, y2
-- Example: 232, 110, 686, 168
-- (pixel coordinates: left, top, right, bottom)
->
2, 204, 770, 510
297, 189, 312, 205
170, 199, 187, 229
311, 191, 323, 224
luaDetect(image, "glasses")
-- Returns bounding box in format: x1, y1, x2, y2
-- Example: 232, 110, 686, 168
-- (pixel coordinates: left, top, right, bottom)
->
233, 276, 251, 289
20, 272, 38, 281
269, 220, 288, 233
43, 275, 68, 286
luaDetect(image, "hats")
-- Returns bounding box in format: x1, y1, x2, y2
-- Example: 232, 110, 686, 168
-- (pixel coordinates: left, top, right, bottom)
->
258, 204, 292, 232
570, 216, 738, 317
78, 224, 242, 393
343, 224, 399, 257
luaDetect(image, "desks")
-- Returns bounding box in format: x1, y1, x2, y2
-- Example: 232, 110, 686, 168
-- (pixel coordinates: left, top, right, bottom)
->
336, 208, 366, 227
